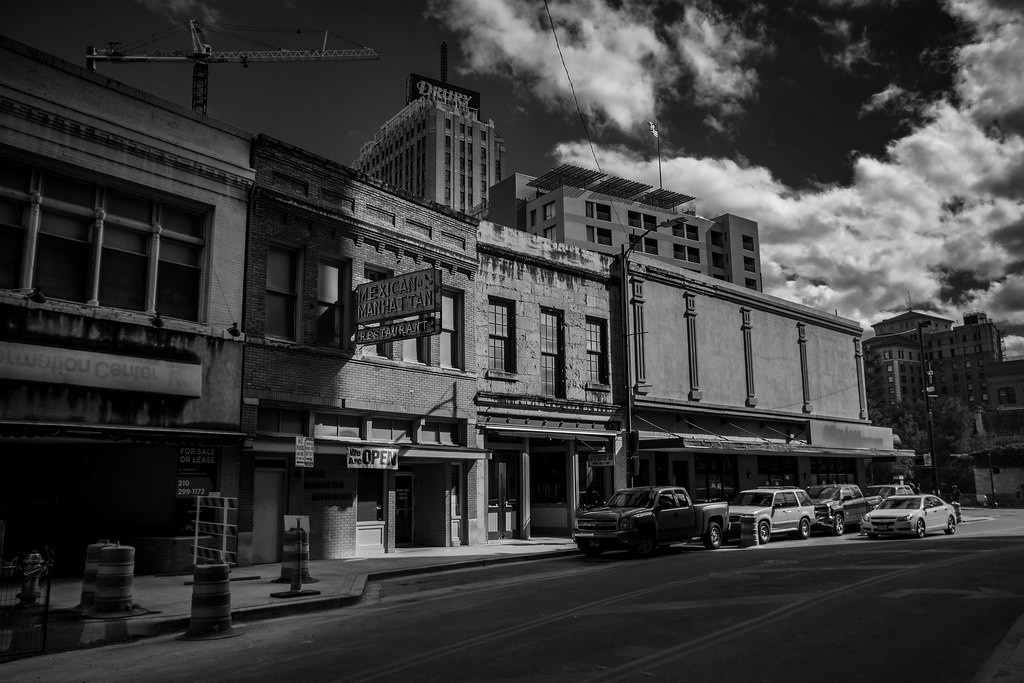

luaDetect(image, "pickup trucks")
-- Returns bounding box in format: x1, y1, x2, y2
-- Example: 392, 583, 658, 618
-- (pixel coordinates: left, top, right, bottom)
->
806, 481, 882, 537
573, 483, 730, 557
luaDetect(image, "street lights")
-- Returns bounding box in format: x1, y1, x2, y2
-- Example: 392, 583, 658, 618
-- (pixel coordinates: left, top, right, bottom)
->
915, 317, 948, 501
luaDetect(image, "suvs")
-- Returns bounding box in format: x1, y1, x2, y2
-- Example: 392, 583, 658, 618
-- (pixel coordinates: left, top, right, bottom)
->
729, 484, 817, 548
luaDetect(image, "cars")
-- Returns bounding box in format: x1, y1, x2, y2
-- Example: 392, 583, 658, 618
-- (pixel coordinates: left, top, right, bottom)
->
859, 492, 956, 538
864, 486, 914, 512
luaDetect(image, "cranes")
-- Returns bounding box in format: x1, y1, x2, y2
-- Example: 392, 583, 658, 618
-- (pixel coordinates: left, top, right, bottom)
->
81, 15, 384, 116
